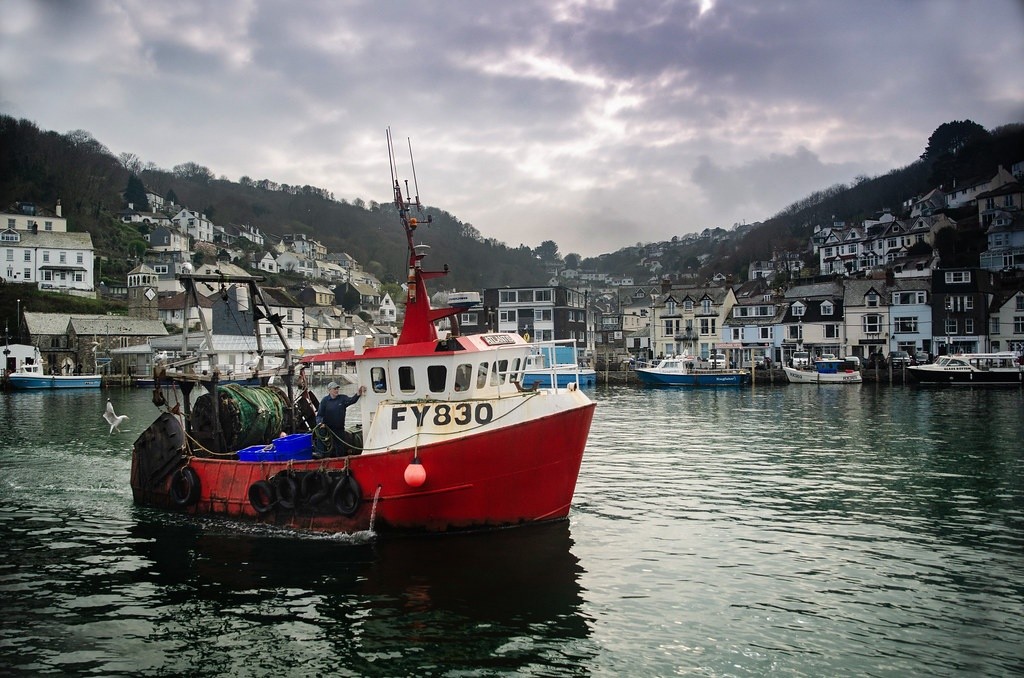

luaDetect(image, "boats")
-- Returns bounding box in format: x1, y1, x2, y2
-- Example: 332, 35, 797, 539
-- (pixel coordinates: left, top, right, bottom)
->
905, 352, 1022, 384
523, 352, 596, 386
784, 357, 864, 386
635, 345, 749, 388
128, 124, 598, 539
3, 344, 103, 390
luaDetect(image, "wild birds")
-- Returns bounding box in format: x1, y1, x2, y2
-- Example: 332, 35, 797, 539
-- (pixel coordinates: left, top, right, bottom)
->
103, 402, 129, 434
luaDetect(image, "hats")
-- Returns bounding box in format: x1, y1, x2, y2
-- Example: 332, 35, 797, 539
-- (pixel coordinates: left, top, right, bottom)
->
327, 382, 340, 390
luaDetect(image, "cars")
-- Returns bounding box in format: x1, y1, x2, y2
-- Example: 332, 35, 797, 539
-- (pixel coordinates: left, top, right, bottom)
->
888, 350, 910, 368
913, 352, 930, 364
819, 354, 837, 361
791, 351, 810, 367
867, 352, 885, 365
707, 352, 731, 369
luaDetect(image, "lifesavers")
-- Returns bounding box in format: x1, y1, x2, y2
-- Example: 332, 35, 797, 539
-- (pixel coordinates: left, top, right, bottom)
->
332, 474, 361, 515
169, 466, 199, 504
300, 470, 329, 506
248, 479, 273, 514
275, 476, 299, 510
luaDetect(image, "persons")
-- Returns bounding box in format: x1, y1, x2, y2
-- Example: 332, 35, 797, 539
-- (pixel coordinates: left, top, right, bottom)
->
312, 381, 366, 460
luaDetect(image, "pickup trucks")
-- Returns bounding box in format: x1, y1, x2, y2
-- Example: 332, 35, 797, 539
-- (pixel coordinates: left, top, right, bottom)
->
742, 356, 774, 370
